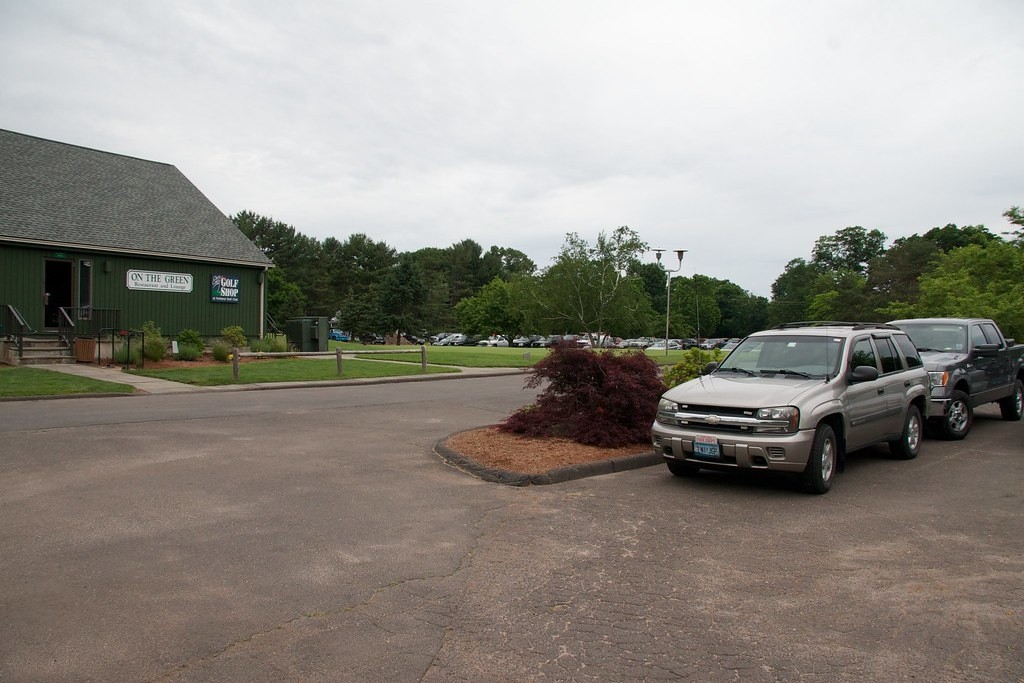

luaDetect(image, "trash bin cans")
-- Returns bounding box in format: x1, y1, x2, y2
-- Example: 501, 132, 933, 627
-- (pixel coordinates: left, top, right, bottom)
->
75, 336, 96, 363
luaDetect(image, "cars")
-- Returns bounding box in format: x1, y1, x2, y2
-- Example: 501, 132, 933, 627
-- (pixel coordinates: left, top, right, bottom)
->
608, 337, 658, 350
403, 335, 425, 345
360, 333, 386, 345
646, 341, 682, 350
720, 338, 742, 349
531, 335, 579, 348
673, 338, 730, 350
514, 335, 544, 348
430, 333, 462, 346
720, 342, 738, 351
478, 335, 509, 347
450, 338, 479, 346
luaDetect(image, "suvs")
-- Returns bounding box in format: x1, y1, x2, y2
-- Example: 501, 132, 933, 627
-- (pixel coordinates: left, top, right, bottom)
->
651, 321, 933, 494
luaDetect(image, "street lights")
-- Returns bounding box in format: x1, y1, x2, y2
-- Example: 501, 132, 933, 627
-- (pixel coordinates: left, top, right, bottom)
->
651, 249, 688, 355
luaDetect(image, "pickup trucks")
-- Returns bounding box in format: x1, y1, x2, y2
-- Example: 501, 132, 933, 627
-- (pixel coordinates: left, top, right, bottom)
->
885, 318, 1024, 440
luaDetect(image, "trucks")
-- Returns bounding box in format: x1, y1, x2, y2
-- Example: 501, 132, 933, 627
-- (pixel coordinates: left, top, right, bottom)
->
576, 332, 611, 348
329, 329, 348, 342
